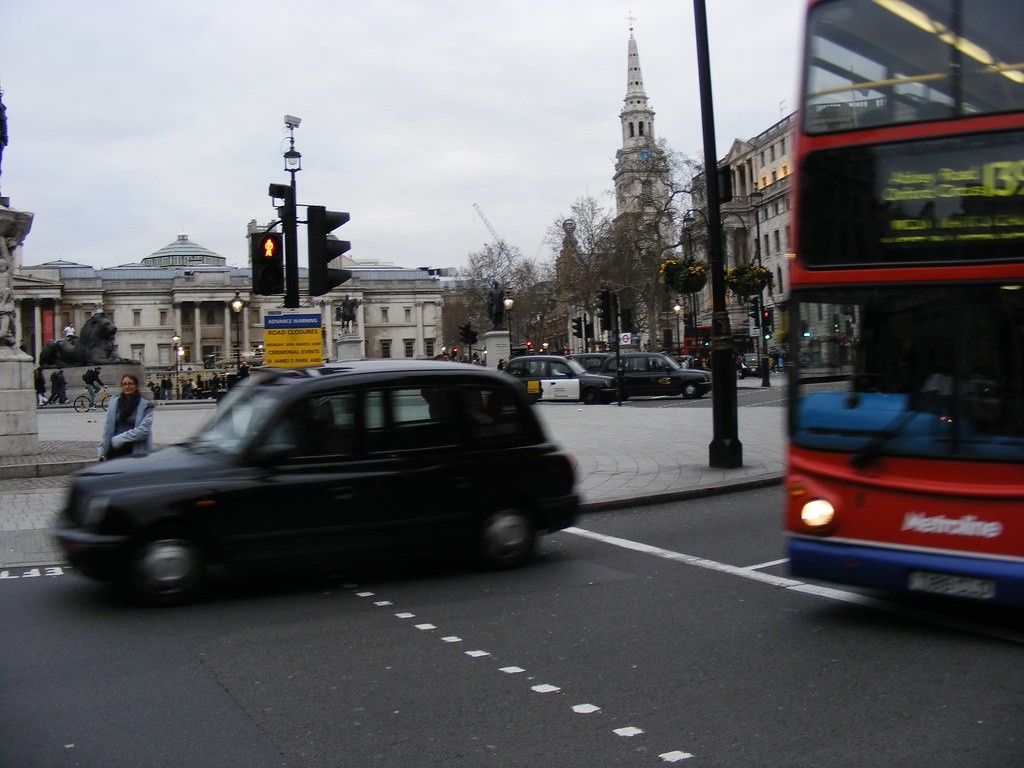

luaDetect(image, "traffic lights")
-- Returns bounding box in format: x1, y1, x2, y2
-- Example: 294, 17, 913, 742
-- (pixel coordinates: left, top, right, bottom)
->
307, 205, 352, 296
749, 296, 855, 339
459, 324, 478, 344
572, 289, 608, 339
452, 348, 457, 356
249, 232, 285, 295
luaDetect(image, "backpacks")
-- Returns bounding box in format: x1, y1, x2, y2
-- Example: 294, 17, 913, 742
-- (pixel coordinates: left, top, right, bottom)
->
81, 370, 94, 382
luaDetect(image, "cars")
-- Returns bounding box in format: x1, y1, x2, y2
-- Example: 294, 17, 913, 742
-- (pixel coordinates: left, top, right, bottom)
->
561, 352, 713, 400
48, 361, 581, 610
738, 348, 812, 380
503, 355, 619, 406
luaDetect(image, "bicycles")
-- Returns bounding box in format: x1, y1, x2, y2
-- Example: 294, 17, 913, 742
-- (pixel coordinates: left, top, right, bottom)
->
73, 385, 113, 413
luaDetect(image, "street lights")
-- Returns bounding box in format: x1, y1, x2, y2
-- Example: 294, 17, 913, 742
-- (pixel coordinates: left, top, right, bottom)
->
229, 288, 245, 370
672, 300, 682, 355
502, 290, 515, 355
178, 345, 183, 371
269, 143, 301, 309
747, 181, 772, 387
172, 332, 181, 400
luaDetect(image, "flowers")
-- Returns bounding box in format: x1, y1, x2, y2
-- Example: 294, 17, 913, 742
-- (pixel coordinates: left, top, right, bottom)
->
658, 258, 709, 287
723, 266, 777, 306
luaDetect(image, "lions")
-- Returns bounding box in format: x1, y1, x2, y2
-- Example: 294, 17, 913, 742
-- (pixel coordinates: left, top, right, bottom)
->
38, 312, 141, 369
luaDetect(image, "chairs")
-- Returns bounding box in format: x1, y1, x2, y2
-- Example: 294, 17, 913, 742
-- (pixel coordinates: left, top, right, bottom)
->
916, 101, 955, 120
856, 109, 893, 126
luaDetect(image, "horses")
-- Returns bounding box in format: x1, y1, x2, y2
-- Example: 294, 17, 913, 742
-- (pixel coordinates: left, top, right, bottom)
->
342, 298, 359, 329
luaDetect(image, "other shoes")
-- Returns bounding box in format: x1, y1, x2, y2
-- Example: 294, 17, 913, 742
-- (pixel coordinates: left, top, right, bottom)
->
89, 407, 96, 410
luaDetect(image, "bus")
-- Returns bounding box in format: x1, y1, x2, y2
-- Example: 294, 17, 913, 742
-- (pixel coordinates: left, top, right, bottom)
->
774, 0, 1021, 614
682, 323, 750, 358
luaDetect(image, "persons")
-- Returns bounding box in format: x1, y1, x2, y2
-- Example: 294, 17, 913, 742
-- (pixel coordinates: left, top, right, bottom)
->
100, 373, 154, 462
84, 367, 108, 411
770, 349, 788, 375
338, 295, 359, 326
148, 367, 219, 401
434, 352, 515, 371
19, 339, 28, 352
240, 361, 250, 378
33, 367, 49, 407
63, 322, 79, 344
49, 368, 70, 405
677, 354, 745, 380
487, 281, 507, 331
